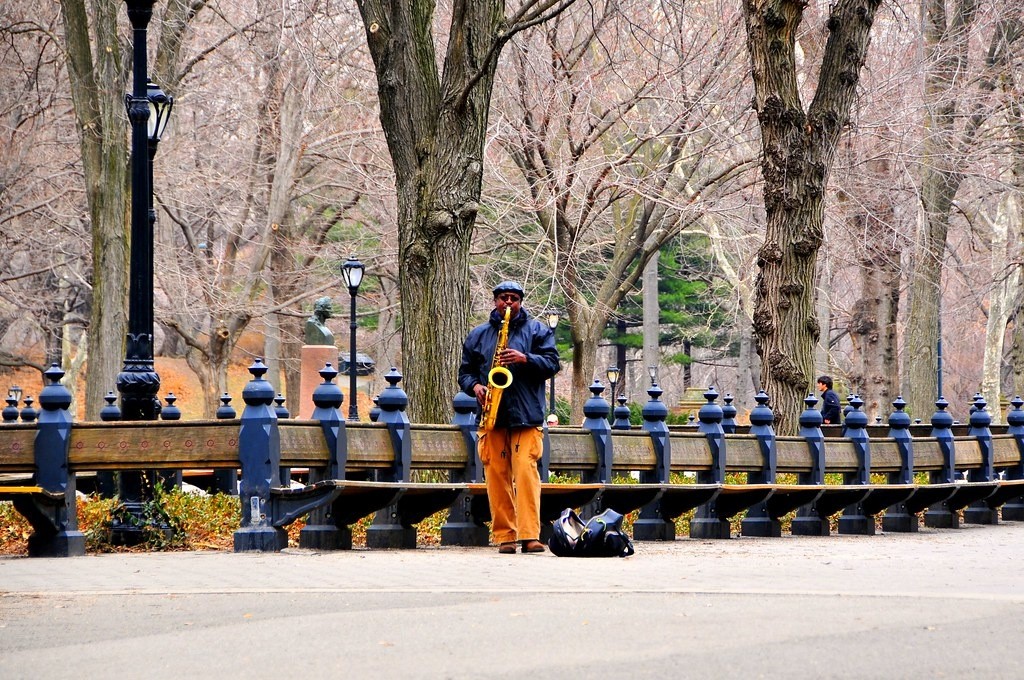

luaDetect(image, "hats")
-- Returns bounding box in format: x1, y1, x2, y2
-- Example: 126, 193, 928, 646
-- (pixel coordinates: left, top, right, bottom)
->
492, 281, 524, 302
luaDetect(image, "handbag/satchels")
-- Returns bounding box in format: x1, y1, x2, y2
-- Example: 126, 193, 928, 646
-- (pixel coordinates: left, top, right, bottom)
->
549, 507, 634, 558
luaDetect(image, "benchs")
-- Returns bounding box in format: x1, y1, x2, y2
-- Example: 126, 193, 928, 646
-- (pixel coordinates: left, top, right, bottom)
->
269, 479, 1024, 528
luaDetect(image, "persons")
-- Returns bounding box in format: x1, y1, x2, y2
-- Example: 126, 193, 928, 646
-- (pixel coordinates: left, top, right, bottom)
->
817, 376, 842, 425
304, 297, 335, 346
457, 282, 561, 553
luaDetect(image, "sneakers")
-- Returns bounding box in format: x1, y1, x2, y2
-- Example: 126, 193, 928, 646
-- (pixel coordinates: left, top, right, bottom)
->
499, 542, 516, 553
521, 540, 545, 553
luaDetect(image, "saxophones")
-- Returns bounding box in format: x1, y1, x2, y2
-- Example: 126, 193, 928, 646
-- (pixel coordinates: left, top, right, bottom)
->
479, 306, 513, 432
